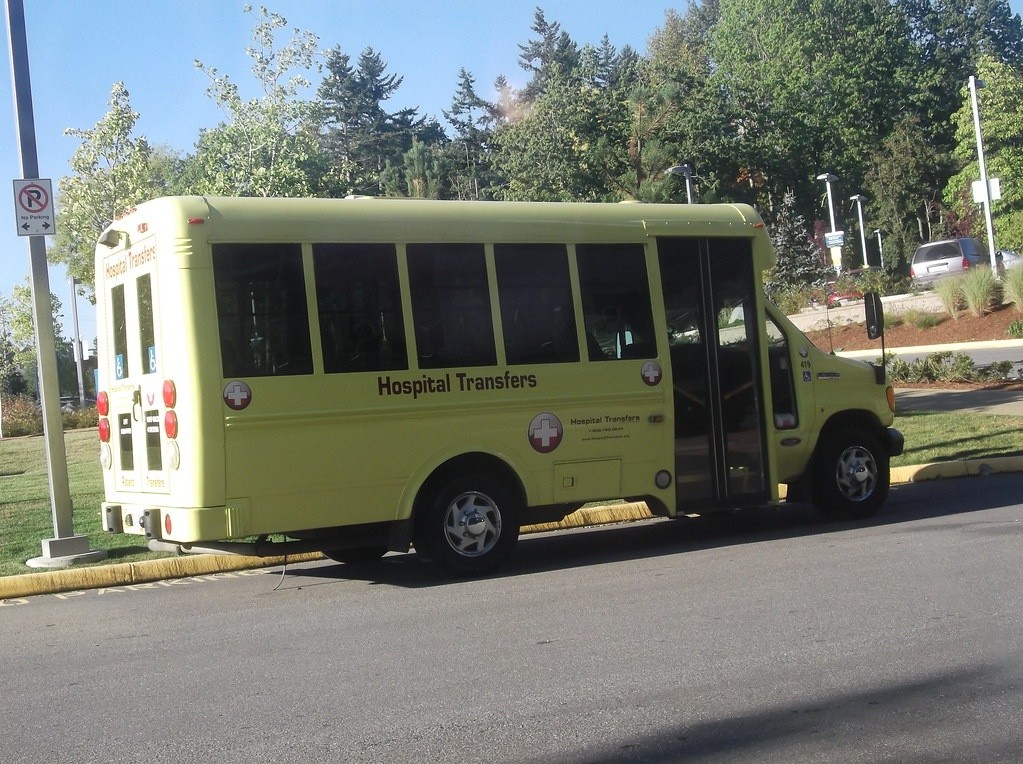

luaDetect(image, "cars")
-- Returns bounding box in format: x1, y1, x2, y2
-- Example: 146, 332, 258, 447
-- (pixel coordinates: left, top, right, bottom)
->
911, 237, 1004, 294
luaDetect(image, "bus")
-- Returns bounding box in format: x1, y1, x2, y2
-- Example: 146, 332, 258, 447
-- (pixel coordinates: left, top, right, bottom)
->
95, 194, 903, 580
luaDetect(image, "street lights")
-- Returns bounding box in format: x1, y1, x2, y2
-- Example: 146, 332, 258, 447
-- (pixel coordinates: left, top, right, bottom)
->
817, 173, 841, 276
849, 194, 868, 270
874, 228, 885, 270
669, 163, 693, 205
